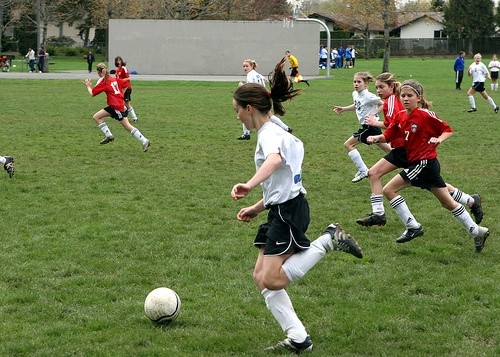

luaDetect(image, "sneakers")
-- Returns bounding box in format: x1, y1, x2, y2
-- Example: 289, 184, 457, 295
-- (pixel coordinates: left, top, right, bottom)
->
352, 169, 369, 183
322, 223, 363, 258
469, 194, 484, 224
494, 105, 500, 113
356, 213, 387, 226
396, 223, 424, 243
237, 135, 250, 140
143, 140, 151, 151
287, 128, 294, 133
4, 156, 15, 178
264, 335, 313, 354
467, 108, 477, 112
100, 135, 115, 145
473, 226, 490, 253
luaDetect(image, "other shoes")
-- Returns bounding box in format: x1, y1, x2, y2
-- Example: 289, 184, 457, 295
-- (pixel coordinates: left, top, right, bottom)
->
133, 118, 141, 121
306, 80, 309, 86
456, 88, 462, 90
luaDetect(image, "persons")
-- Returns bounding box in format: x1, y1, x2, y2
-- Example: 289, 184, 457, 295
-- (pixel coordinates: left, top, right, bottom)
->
467, 53, 499, 113
24, 47, 36, 73
237, 60, 293, 140
0, 55, 8, 68
488, 54, 500, 92
114, 56, 138, 123
334, 72, 394, 182
37, 47, 46, 74
355, 73, 491, 254
320, 44, 356, 69
230, 60, 363, 353
453, 51, 466, 90
84, 63, 150, 151
0, 156, 14, 177
285, 51, 310, 88
84, 50, 95, 73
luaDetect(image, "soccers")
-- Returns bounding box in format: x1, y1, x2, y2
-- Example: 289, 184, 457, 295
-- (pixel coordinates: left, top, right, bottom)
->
143, 287, 182, 325
